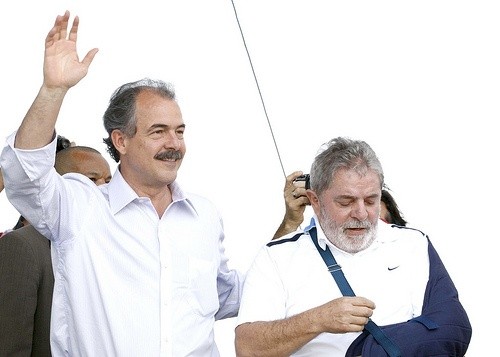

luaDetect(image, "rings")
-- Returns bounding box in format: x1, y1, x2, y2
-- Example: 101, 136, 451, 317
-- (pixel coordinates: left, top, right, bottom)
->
291, 192, 297, 198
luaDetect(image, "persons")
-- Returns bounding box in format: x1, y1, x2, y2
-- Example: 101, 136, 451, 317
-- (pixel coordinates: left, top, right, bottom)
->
0, 146, 113, 357
271, 165, 406, 240
0, 133, 73, 236
0, 9, 244, 357
231, 136, 473, 357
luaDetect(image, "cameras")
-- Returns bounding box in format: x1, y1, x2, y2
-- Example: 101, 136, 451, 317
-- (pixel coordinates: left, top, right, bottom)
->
295, 174, 311, 205
